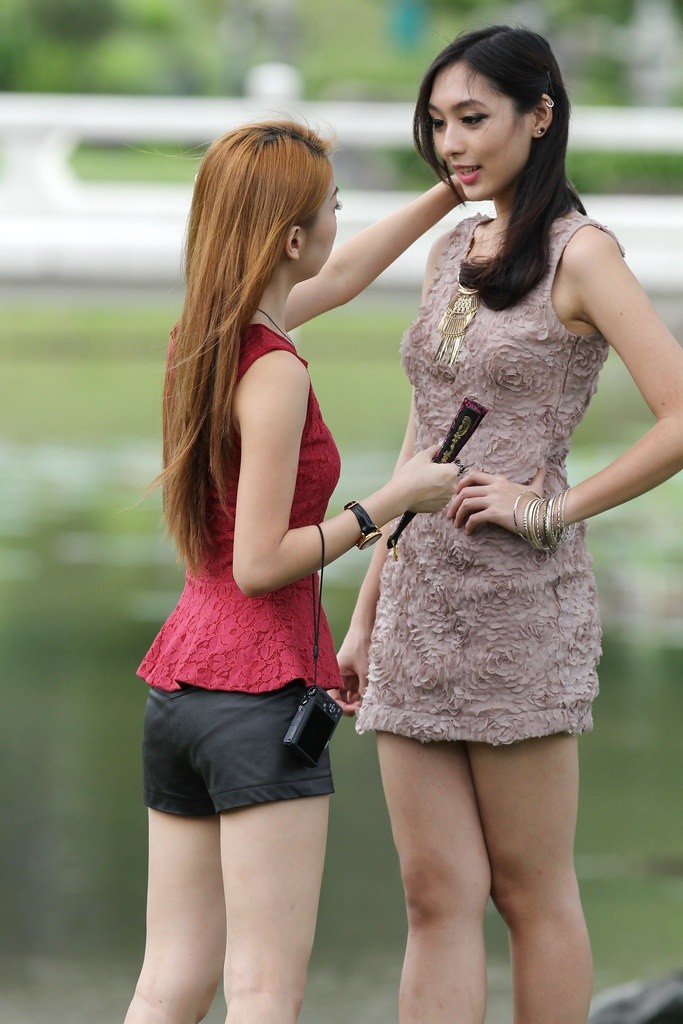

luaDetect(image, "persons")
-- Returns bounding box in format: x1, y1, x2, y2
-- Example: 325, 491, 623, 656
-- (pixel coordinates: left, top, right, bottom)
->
123, 116, 469, 1024
326, 24, 683, 1024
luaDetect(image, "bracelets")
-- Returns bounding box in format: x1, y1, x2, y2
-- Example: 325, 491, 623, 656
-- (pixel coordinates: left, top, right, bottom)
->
512, 489, 577, 551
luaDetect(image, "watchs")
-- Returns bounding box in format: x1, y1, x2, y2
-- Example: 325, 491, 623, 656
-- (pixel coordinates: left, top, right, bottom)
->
344, 500, 383, 549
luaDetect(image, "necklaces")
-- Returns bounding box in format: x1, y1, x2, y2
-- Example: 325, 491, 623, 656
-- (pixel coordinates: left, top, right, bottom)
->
258, 308, 296, 347
435, 266, 477, 364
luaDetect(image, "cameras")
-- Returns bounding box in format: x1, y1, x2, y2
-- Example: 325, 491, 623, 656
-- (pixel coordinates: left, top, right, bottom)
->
283, 686, 344, 768
